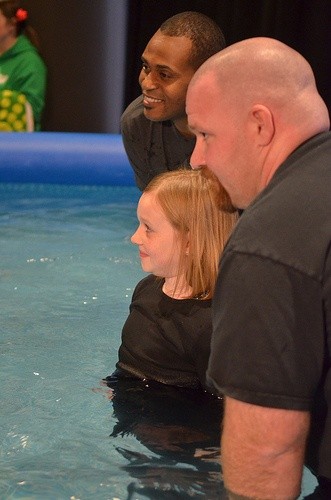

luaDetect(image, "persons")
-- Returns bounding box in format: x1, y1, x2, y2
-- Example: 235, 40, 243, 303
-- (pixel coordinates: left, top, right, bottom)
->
184, 34, 331, 500
0, 0, 47, 134
107, 166, 242, 388
118, 11, 227, 191
